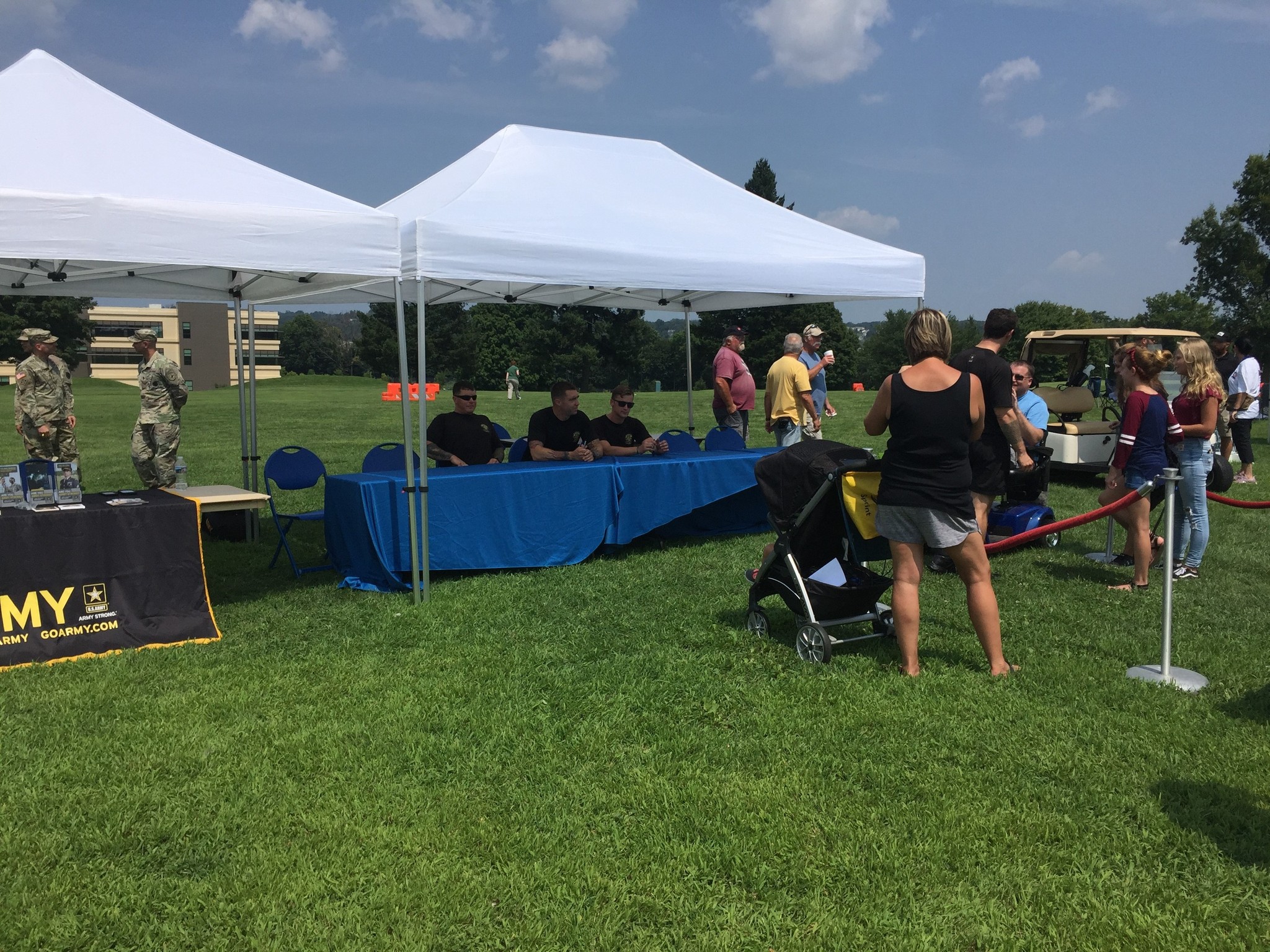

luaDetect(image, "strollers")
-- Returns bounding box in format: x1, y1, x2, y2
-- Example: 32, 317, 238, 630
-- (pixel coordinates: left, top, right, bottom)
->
745, 440, 918, 666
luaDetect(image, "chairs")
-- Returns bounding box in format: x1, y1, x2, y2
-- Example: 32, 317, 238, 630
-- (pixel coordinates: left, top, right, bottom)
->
1099, 379, 1118, 408
1087, 376, 1102, 408
705, 426, 747, 450
1007, 429, 1054, 501
658, 430, 701, 451
264, 446, 333, 578
362, 443, 420, 472
508, 436, 528, 462
492, 422, 511, 439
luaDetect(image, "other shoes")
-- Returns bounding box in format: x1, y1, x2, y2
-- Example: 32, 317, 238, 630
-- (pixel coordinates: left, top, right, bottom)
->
745, 569, 758, 583
508, 399, 511, 400
517, 397, 521, 400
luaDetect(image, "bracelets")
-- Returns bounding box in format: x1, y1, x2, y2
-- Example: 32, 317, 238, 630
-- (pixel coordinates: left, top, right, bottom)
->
765, 418, 770, 421
637, 446, 639, 454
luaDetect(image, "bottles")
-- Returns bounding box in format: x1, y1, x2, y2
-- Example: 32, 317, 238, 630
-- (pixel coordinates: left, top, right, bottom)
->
175, 456, 188, 491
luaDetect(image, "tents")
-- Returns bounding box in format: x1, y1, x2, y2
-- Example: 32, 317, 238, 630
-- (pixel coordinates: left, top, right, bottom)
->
0, 48, 420, 606
247, 125, 928, 608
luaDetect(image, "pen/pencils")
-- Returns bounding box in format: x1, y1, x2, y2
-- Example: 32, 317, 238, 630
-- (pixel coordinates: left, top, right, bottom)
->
583, 441, 586, 449
652, 439, 655, 457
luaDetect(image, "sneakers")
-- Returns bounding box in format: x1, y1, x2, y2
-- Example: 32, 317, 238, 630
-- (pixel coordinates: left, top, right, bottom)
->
927, 555, 956, 573
1154, 563, 1163, 570
1234, 472, 1256, 485
1173, 567, 1200, 581
1110, 553, 1135, 567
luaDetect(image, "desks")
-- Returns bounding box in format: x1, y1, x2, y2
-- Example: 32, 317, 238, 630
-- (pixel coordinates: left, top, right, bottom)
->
323, 447, 787, 593
0, 485, 265, 671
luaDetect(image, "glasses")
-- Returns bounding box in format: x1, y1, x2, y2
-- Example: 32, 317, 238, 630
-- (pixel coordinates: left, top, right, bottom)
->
1014, 374, 1029, 381
454, 395, 477, 400
614, 400, 634, 408
728, 336, 745, 340
805, 325, 817, 334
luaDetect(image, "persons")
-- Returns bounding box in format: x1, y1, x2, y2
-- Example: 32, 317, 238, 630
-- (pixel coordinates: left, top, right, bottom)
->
947, 307, 1035, 547
506, 360, 521, 401
526, 382, 603, 462
0, 476, 21, 493
764, 333, 820, 447
711, 325, 755, 450
419, 380, 506, 468
1097, 329, 1261, 593
28, 472, 47, 490
590, 385, 669, 457
13, 328, 82, 486
864, 309, 1022, 677
127, 328, 188, 490
798, 324, 836, 440
1009, 359, 1050, 474
60, 466, 80, 489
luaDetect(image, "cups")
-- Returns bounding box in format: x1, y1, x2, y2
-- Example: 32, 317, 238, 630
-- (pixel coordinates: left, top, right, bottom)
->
824, 349, 836, 365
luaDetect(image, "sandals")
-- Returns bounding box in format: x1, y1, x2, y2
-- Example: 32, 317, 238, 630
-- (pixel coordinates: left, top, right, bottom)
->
1149, 536, 1164, 565
1115, 580, 1150, 591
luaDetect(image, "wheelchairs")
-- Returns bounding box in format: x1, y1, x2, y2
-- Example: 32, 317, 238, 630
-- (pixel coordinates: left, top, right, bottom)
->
919, 427, 1061, 572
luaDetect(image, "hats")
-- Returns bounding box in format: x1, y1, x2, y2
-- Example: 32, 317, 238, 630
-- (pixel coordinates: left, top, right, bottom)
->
722, 325, 748, 338
803, 325, 827, 337
27, 329, 59, 343
127, 329, 157, 342
16, 328, 40, 341
1212, 331, 1232, 343
62, 466, 72, 471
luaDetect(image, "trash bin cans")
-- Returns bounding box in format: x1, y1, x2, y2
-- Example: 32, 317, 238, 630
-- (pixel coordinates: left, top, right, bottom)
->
655, 381, 661, 392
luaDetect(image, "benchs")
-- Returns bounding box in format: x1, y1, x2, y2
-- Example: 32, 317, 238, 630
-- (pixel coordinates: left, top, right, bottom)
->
1032, 386, 1121, 434
